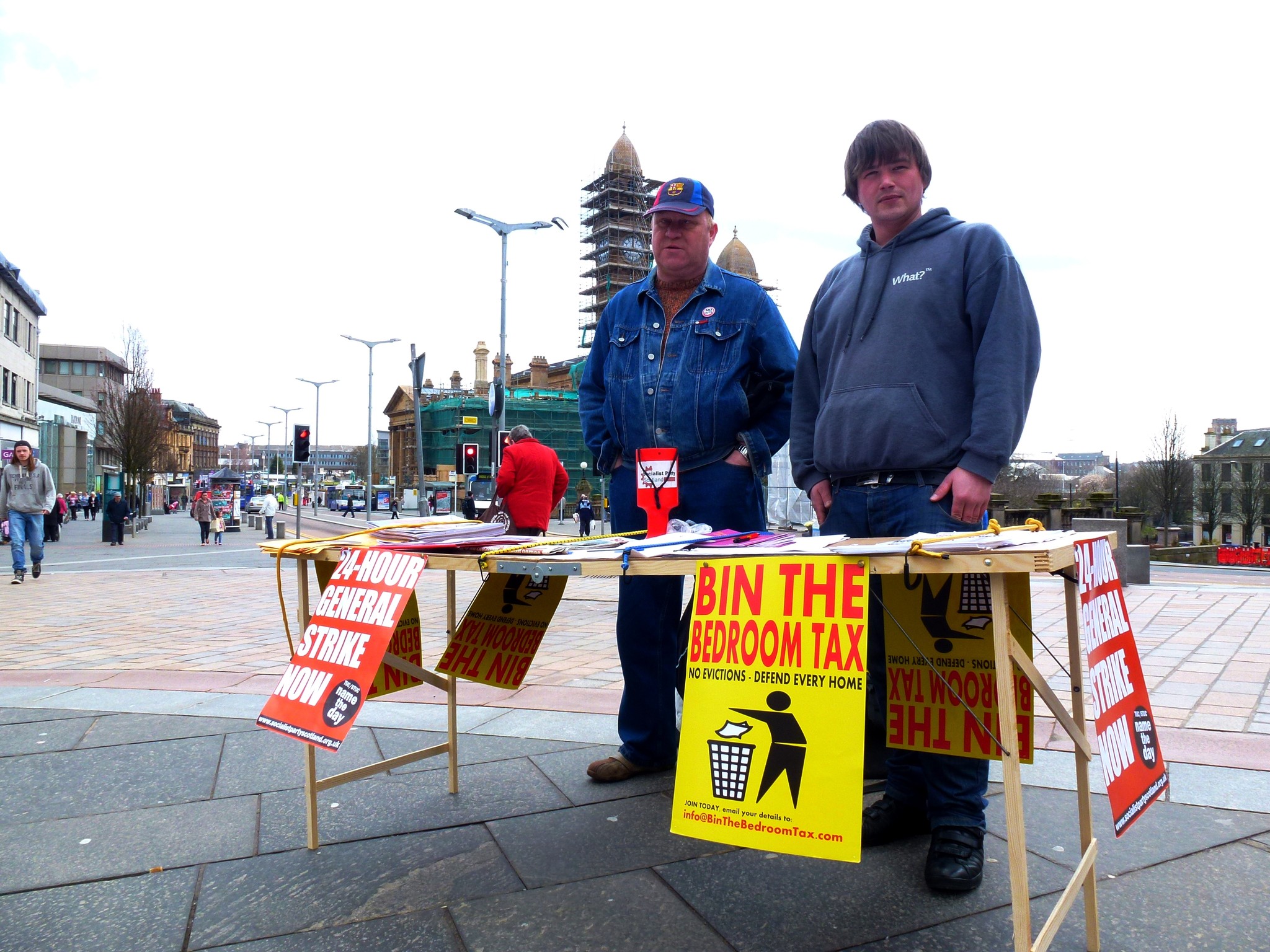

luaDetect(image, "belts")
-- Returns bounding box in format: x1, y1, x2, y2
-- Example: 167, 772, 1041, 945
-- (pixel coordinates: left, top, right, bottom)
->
832, 468, 948, 485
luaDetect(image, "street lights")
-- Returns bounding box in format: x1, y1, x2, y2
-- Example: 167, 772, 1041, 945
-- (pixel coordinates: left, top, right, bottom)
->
269, 406, 303, 511
241, 434, 265, 485
340, 335, 401, 521
576, 462, 592, 514
454, 206, 568, 506
296, 378, 340, 516
256, 421, 283, 489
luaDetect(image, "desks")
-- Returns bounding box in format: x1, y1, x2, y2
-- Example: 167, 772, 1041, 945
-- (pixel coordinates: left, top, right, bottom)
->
269, 529, 1117, 952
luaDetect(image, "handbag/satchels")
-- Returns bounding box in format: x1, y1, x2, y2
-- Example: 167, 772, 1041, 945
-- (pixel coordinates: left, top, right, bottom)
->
210, 518, 220, 532
63, 514, 69, 524
590, 519, 596, 530
573, 513, 580, 523
478, 489, 517, 536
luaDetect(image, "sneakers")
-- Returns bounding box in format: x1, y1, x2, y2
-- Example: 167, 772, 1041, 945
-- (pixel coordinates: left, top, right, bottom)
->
11, 570, 24, 583
924, 825, 984, 890
587, 751, 676, 781
32, 561, 41, 579
861, 793, 929, 847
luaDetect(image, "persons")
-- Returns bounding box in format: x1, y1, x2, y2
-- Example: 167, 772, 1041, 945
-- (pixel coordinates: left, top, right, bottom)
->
342, 494, 354, 518
0, 441, 56, 584
164, 501, 178, 514
464, 491, 476, 520
495, 423, 569, 536
124, 493, 140, 508
787, 121, 1041, 895
302, 496, 309, 506
106, 492, 130, 546
575, 494, 595, 537
400, 496, 405, 505
390, 497, 399, 519
578, 177, 800, 781
428, 494, 433, 501
428, 497, 434, 508
194, 491, 226, 546
317, 496, 321, 507
44, 491, 103, 543
278, 492, 288, 510
557, 496, 566, 520
604, 495, 610, 523
259, 489, 278, 539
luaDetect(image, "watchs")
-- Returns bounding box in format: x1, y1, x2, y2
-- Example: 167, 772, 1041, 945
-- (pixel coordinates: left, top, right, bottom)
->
735, 444, 751, 463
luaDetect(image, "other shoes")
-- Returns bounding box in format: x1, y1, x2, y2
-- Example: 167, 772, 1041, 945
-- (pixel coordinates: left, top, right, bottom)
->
206, 539, 209, 544
219, 542, 222, 545
119, 542, 123, 545
587, 535, 589, 536
111, 542, 116, 545
580, 532, 583, 537
351, 516, 355, 518
201, 543, 205, 546
342, 514, 346, 517
71, 518, 95, 521
266, 537, 274, 539
215, 541, 217, 544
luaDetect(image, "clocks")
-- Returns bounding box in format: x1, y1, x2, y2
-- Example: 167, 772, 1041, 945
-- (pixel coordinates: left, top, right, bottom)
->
597, 239, 611, 262
620, 233, 645, 265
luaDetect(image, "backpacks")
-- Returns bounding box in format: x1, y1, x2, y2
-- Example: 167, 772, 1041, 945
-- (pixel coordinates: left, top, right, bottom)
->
462, 501, 468, 514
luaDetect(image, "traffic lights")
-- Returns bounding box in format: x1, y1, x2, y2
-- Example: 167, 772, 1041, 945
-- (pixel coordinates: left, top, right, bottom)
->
498, 430, 511, 467
463, 443, 478, 475
293, 424, 310, 464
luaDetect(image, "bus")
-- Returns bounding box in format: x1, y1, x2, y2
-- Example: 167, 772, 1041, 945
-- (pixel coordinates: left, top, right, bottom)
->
235, 471, 292, 509
464, 471, 498, 520
327, 485, 365, 511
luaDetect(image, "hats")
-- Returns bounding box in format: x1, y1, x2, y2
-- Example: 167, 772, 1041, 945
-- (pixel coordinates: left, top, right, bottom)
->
115, 492, 121, 496
57, 493, 63, 498
643, 177, 714, 220
14, 440, 31, 448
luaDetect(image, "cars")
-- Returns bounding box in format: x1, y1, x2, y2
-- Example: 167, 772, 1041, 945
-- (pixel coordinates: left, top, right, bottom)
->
245, 497, 265, 514
1217, 544, 1254, 551
1178, 542, 1196, 547
